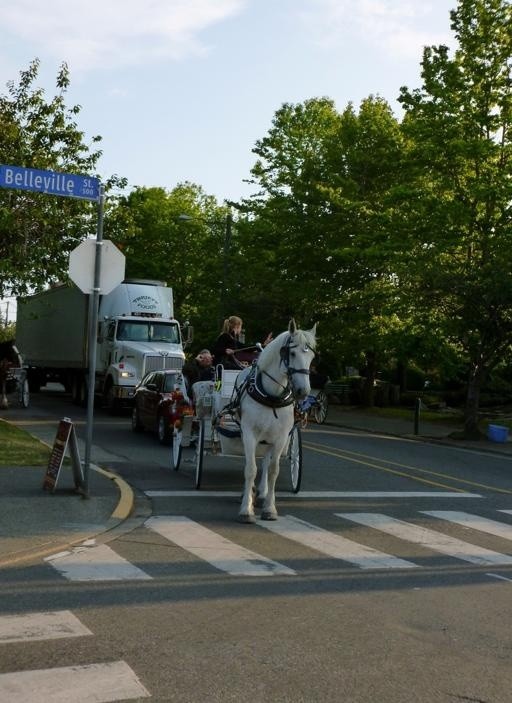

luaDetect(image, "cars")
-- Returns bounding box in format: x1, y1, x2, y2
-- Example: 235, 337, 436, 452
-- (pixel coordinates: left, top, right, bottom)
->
132, 369, 189, 443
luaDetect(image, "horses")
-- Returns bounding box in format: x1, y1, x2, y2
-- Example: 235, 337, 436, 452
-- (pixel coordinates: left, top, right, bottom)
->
235, 317, 320, 524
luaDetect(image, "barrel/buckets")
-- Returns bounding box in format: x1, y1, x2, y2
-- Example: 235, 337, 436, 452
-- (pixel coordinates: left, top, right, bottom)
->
488, 424, 507, 443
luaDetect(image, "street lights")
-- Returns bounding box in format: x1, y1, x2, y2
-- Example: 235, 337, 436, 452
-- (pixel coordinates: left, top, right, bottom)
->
178, 214, 232, 335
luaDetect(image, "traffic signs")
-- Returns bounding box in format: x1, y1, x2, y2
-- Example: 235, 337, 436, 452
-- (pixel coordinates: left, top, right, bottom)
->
1, 166, 100, 200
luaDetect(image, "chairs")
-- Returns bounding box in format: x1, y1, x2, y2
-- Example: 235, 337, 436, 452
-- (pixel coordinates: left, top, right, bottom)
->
222, 367, 241, 397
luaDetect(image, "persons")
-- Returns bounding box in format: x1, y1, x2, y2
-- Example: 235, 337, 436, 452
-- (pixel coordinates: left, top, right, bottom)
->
0, 352, 14, 366
181, 347, 216, 416
295, 361, 329, 429
214, 315, 274, 370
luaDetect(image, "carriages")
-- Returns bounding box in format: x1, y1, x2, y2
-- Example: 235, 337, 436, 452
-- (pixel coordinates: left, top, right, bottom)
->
171, 317, 328, 524
0, 339, 30, 409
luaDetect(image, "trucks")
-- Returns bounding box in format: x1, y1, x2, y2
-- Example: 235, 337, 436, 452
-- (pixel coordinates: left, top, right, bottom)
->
15, 279, 194, 414
